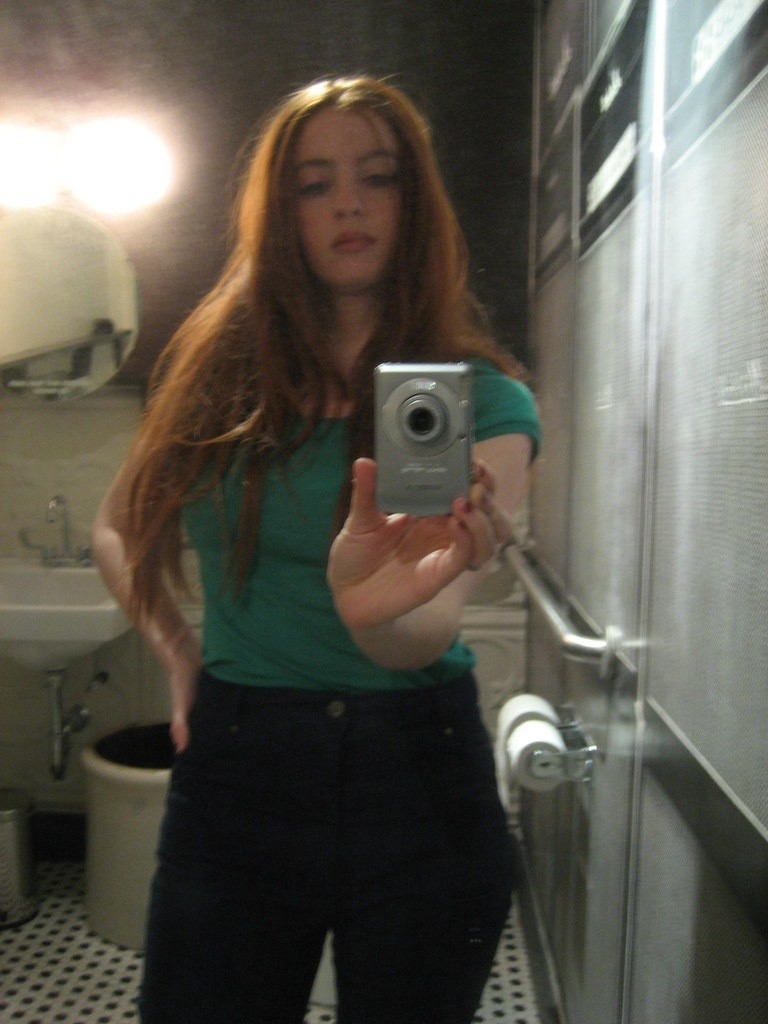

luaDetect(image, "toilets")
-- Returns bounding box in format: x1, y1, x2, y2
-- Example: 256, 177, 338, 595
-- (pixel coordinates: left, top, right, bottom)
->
301, 603, 529, 1008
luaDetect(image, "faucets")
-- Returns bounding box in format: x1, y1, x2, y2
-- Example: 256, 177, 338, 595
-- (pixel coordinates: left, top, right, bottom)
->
46, 496, 74, 559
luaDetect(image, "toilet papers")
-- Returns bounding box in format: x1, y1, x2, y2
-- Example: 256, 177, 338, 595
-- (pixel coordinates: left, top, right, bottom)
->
494, 694, 568, 811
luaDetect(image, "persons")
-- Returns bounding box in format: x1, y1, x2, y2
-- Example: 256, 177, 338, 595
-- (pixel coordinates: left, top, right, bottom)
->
92, 78, 543, 1024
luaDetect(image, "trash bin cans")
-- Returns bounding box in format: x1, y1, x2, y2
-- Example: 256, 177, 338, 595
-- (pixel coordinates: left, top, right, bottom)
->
0, 787, 42, 930
81, 717, 178, 952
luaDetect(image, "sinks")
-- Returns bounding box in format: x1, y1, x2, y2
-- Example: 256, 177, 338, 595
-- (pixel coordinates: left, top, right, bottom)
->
0, 557, 137, 673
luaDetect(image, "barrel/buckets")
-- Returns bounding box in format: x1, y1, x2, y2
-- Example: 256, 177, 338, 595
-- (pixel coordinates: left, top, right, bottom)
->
82, 722, 187, 951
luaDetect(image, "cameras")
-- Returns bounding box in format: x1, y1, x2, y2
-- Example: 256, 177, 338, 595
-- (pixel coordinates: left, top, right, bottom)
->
371, 360, 472, 518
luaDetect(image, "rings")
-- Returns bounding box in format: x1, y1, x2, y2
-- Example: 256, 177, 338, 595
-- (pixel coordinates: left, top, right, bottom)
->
467, 564, 480, 572
